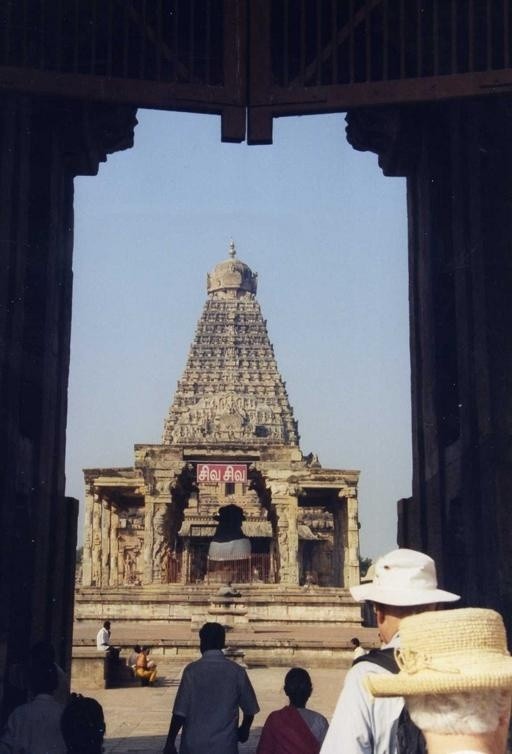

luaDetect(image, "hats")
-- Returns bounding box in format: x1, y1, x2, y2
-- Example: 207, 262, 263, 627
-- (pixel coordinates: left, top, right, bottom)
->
367, 608, 511, 696
349, 549, 460, 607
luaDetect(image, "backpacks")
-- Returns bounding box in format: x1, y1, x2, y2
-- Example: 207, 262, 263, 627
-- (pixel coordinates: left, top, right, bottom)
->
351, 648, 426, 753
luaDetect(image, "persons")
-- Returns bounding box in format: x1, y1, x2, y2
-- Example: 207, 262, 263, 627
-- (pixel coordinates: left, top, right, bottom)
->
363, 606, 512, 754
162, 622, 260, 754
319, 548, 461, 754
350, 637, 370, 662
0, 646, 70, 754
96, 621, 115, 658
128, 644, 157, 686
255, 667, 329, 754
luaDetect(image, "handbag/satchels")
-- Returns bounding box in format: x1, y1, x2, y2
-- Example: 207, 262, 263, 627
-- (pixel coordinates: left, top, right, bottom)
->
61, 694, 106, 754
